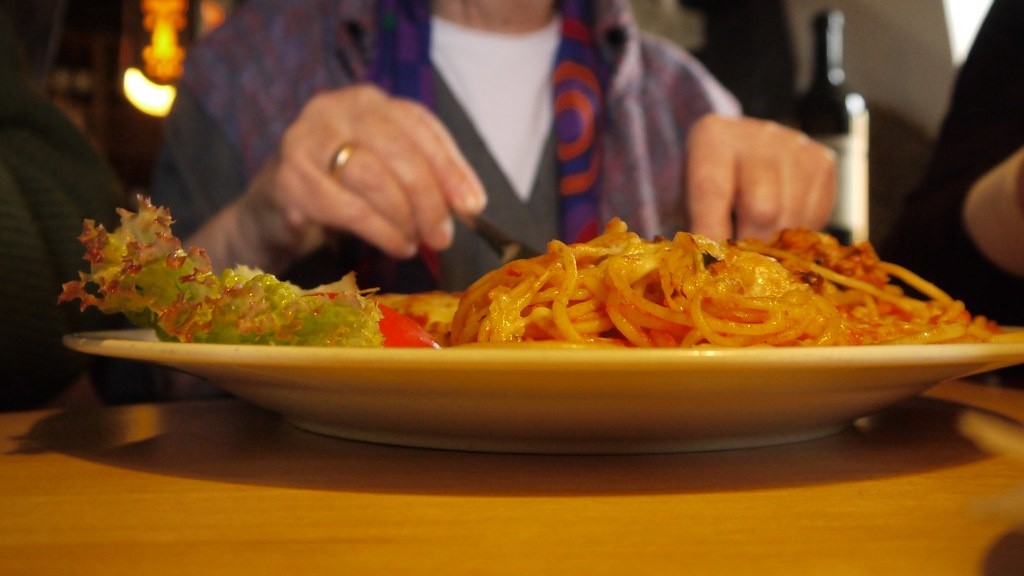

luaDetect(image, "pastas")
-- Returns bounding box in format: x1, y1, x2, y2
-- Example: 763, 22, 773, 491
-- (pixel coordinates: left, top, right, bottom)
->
450, 219, 1001, 346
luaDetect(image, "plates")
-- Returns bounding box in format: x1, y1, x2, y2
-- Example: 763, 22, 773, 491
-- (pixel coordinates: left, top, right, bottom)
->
60, 291, 1023, 452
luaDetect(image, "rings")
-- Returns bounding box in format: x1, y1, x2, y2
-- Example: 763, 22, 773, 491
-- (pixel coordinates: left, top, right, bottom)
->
329, 141, 357, 184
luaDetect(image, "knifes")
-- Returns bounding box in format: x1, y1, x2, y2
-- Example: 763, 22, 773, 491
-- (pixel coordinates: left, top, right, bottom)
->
335, 19, 537, 264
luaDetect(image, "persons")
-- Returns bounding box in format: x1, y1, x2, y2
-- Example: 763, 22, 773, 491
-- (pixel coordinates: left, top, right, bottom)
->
147, 0, 844, 295
875, 0, 1024, 391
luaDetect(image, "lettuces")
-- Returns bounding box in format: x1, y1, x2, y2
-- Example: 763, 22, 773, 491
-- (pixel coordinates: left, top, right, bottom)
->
61, 197, 385, 350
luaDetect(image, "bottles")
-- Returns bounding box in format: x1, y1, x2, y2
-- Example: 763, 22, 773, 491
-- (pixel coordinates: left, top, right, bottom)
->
802, 11, 870, 247
